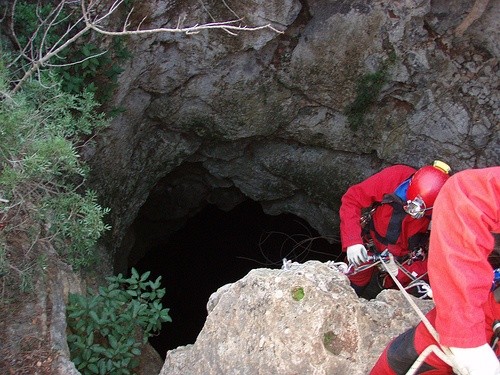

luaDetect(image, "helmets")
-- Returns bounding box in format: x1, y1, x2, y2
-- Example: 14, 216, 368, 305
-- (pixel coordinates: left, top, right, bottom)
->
406, 166, 450, 219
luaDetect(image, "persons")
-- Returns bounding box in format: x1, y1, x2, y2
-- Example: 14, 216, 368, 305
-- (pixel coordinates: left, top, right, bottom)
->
369, 166, 500, 375
339, 161, 451, 302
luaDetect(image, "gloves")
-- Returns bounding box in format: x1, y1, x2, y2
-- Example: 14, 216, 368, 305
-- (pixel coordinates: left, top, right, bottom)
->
381, 253, 398, 277
347, 243, 368, 269
448, 342, 500, 375
